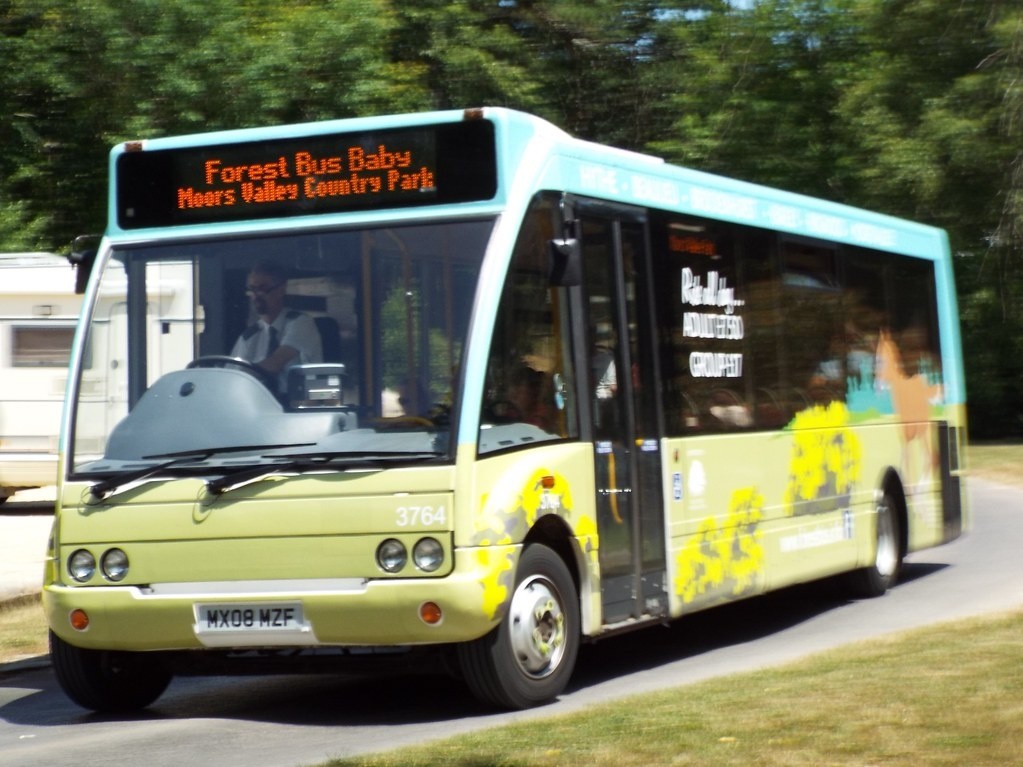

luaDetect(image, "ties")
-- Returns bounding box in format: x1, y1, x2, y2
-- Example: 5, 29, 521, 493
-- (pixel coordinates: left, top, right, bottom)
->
267, 327, 278, 358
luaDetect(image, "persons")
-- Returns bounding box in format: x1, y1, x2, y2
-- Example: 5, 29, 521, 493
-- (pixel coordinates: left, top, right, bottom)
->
503, 367, 550, 429
225, 260, 324, 394
593, 348, 617, 399
397, 376, 427, 415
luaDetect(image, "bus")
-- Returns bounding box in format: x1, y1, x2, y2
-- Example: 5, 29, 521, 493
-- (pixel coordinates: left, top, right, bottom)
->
42, 106, 970, 716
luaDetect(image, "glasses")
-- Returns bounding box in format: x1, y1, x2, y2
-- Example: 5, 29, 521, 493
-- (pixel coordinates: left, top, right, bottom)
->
246, 283, 281, 295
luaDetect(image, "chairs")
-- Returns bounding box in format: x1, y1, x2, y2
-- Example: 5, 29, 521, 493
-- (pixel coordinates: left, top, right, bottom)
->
700, 388, 747, 434
664, 389, 702, 437
812, 386, 843, 425
780, 386, 814, 428
745, 385, 789, 430
